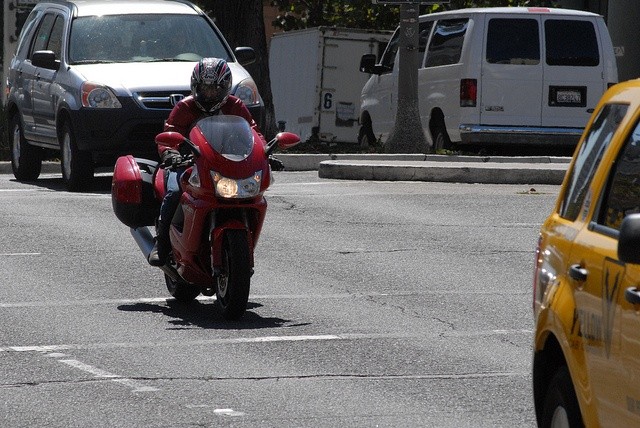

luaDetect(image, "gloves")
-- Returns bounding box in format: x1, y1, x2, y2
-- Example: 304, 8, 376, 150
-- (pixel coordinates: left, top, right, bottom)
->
268, 154, 285, 172
159, 149, 193, 168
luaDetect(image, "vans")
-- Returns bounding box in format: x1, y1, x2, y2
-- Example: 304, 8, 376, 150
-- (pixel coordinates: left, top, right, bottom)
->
356, 6, 620, 153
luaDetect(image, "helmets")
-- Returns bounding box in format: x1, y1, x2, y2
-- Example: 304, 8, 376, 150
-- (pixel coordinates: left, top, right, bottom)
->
190, 56, 232, 115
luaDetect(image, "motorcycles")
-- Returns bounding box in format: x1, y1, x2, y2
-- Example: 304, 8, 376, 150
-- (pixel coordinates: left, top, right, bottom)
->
112, 114, 302, 317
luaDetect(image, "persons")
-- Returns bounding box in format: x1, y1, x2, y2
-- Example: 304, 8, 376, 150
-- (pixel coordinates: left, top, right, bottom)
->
147, 57, 283, 267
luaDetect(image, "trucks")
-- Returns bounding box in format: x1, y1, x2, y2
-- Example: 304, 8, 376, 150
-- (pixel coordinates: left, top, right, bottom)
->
270, 27, 397, 147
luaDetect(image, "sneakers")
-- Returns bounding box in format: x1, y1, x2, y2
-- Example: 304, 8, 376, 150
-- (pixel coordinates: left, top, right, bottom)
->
148, 236, 170, 266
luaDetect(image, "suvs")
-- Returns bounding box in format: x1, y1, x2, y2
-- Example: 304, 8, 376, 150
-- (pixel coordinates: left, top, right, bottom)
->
4, 1, 265, 192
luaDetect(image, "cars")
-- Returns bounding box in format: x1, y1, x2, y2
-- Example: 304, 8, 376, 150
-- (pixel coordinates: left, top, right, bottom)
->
530, 75, 640, 427
